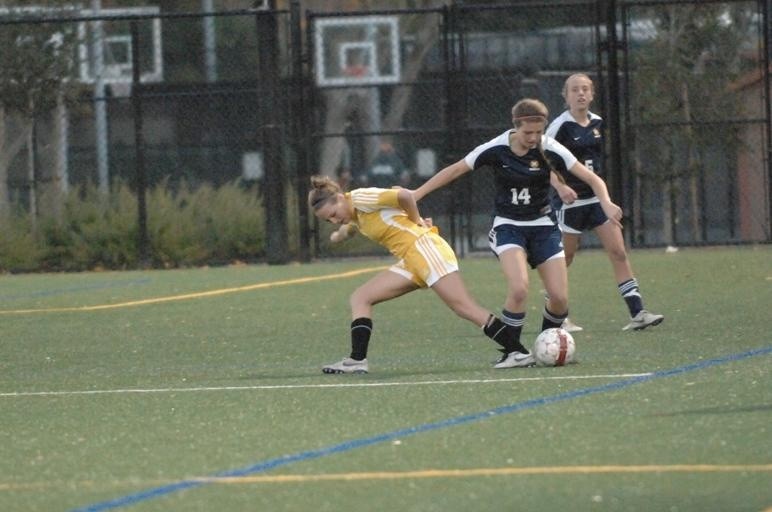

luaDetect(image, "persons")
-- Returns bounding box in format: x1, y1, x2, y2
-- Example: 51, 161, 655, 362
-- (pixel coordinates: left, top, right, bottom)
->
391, 99, 624, 365
308, 175, 536, 374
545, 73, 664, 332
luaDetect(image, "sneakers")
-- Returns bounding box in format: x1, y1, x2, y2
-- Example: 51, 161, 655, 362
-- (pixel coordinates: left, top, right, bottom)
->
628, 309, 665, 332
492, 349, 536, 369
320, 356, 369, 375
559, 317, 584, 333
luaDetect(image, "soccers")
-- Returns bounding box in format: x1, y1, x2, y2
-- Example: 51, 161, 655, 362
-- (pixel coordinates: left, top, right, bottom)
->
534, 328, 575, 366
